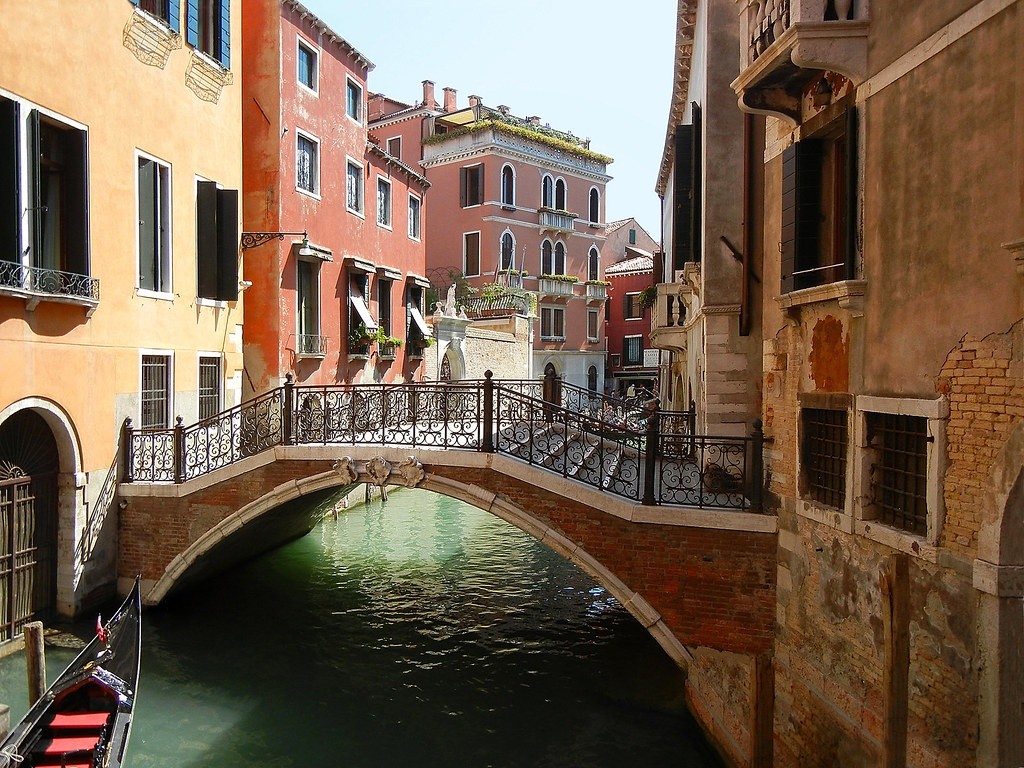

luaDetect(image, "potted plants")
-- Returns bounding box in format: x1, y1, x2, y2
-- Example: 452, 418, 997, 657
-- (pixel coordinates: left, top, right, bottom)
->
385, 336, 402, 348
417, 339, 433, 348
379, 326, 385, 351
356, 325, 376, 345
349, 330, 359, 353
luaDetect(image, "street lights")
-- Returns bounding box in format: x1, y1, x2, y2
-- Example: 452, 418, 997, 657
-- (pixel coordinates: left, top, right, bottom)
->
239, 230, 313, 258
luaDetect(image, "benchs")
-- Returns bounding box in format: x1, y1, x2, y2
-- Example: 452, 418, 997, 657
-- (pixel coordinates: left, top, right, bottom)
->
34, 759, 94, 768
44, 711, 109, 728
30, 737, 99, 755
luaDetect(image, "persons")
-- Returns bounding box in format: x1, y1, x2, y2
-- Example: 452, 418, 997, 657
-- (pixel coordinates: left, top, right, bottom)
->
627, 384, 636, 398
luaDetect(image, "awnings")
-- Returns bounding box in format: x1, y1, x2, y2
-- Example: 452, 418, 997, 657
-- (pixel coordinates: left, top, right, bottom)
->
351, 278, 378, 329
411, 298, 432, 336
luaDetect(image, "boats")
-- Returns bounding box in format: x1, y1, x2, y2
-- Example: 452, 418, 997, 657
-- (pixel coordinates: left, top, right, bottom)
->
0, 571, 143, 768
